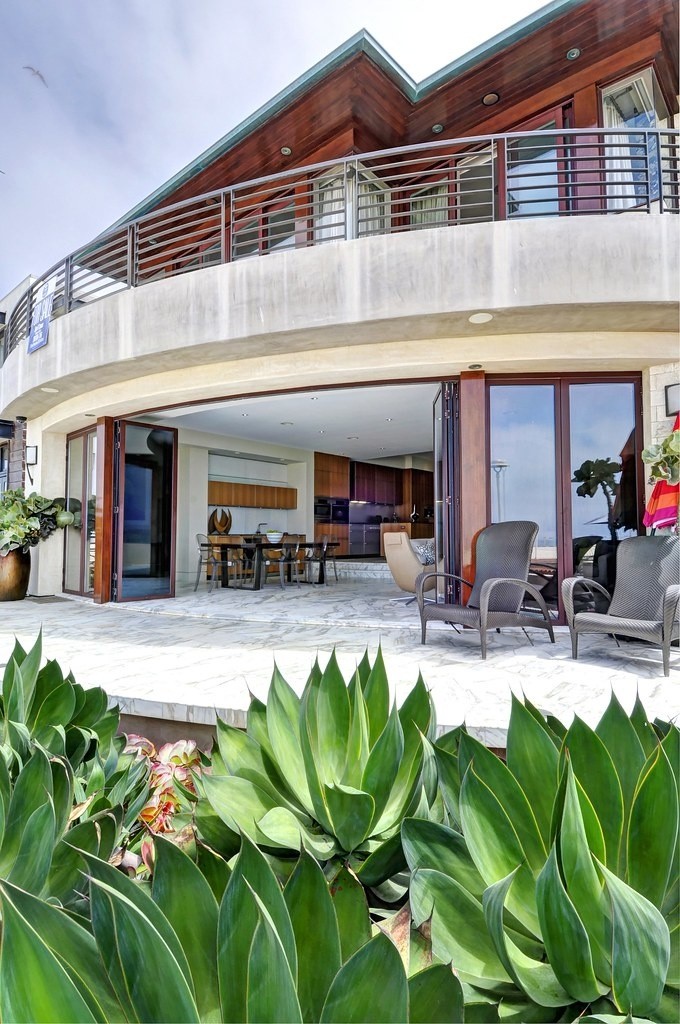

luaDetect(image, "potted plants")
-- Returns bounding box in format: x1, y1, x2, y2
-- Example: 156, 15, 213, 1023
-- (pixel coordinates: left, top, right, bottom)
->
266, 529, 283, 543
571, 456, 623, 614
0, 487, 74, 601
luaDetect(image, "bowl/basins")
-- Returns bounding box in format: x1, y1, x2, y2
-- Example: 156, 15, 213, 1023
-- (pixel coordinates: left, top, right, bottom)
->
266, 533, 284, 544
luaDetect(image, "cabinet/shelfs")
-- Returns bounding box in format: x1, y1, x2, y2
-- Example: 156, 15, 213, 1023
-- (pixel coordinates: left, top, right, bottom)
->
208, 481, 297, 510
314, 451, 434, 557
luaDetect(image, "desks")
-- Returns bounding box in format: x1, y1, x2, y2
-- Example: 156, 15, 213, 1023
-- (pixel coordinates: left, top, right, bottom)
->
201, 543, 340, 590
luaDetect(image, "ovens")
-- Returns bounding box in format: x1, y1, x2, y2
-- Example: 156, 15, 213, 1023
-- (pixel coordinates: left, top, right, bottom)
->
315, 496, 349, 524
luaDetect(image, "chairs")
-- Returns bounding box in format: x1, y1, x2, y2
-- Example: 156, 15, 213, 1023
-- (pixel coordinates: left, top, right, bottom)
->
561, 536, 680, 677
383, 532, 436, 606
415, 521, 555, 660
194, 534, 338, 593
523, 535, 604, 613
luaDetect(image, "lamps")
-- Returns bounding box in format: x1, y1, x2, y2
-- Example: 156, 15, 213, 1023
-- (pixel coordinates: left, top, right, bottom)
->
26, 446, 37, 465
